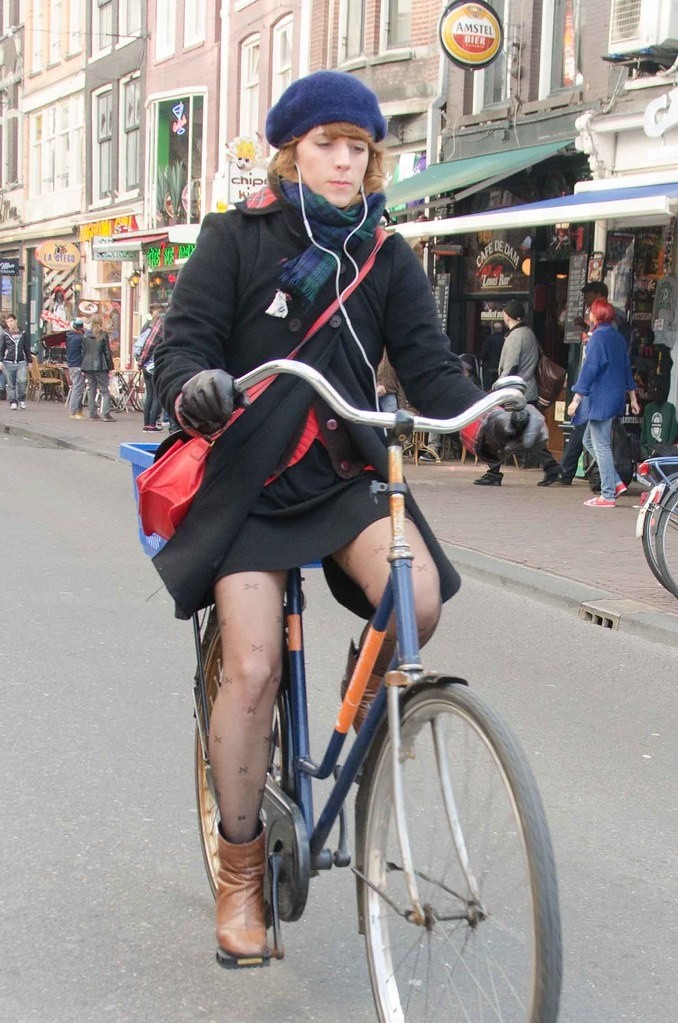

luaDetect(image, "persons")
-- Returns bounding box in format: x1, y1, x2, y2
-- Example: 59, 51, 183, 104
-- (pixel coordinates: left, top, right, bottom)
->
133, 302, 169, 432
152, 70, 550, 959
66, 318, 87, 420
481, 322, 504, 390
567, 299, 639, 507
420, 353, 480, 461
0, 312, 33, 409
473, 299, 562, 486
80, 314, 116, 422
378, 347, 399, 412
557, 280, 631, 491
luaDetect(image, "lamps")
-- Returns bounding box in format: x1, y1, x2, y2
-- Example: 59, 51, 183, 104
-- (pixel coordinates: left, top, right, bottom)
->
155, 271, 164, 285
415, 214, 433, 249
76, 274, 86, 290
129, 278, 137, 288
132, 265, 149, 285
149, 274, 157, 289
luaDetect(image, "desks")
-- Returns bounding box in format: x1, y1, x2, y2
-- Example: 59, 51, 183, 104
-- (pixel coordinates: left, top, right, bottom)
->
37, 362, 68, 402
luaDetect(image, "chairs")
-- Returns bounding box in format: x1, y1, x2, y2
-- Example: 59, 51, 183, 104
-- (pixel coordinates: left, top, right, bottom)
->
26, 352, 66, 402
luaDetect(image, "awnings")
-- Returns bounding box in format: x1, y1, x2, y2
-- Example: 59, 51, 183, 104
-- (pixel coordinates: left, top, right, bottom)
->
386, 183, 678, 238
91, 233, 168, 252
382, 139, 573, 222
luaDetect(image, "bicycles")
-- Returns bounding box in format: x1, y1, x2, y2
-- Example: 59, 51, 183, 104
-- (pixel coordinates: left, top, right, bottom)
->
635, 456, 678, 600
189, 361, 562, 1023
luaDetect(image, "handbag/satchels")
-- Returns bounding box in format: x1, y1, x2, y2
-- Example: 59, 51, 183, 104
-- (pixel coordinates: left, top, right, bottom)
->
137, 437, 213, 541
534, 355, 566, 412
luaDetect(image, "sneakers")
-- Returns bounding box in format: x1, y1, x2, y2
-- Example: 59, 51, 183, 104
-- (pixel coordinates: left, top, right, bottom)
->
615, 483, 626, 497
584, 496, 616, 508
143, 424, 164, 432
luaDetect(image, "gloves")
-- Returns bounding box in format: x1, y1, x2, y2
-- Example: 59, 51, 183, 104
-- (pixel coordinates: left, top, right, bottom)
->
180, 369, 251, 423
486, 404, 549, 455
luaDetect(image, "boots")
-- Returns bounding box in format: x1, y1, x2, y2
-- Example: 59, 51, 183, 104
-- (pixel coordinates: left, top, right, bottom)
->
342, 638, 385, 735
216, 818, 266, 956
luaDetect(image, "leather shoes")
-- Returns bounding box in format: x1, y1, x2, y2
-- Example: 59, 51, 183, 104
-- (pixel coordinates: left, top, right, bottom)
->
472, 470, 502, 486
537, 465, 562, 486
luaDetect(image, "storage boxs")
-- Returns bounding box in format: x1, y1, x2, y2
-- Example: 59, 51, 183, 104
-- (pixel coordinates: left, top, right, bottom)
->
118, 442, 321, 570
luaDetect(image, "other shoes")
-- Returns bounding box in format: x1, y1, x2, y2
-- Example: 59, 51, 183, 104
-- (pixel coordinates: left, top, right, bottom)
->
89, 414, 103, 421
557, 473, 571, 485
20, 400, 26, 408
104, 415, 116, 421
419, 452, 440, 461
10, 402, 18, 409
71, 413, 88, 421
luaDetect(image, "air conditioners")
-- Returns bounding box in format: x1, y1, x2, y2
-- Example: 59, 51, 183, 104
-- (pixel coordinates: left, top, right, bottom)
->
605, 0, 678, 55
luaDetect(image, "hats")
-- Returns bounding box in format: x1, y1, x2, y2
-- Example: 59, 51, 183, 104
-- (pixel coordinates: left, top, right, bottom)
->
266, 70, 386, 148
581, 281, 608, 296
73, 318, 84, 329
502, 299, 525, 321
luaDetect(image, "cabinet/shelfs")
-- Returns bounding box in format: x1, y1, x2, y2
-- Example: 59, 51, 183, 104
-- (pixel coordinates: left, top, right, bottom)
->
110, 370, 148, 412
